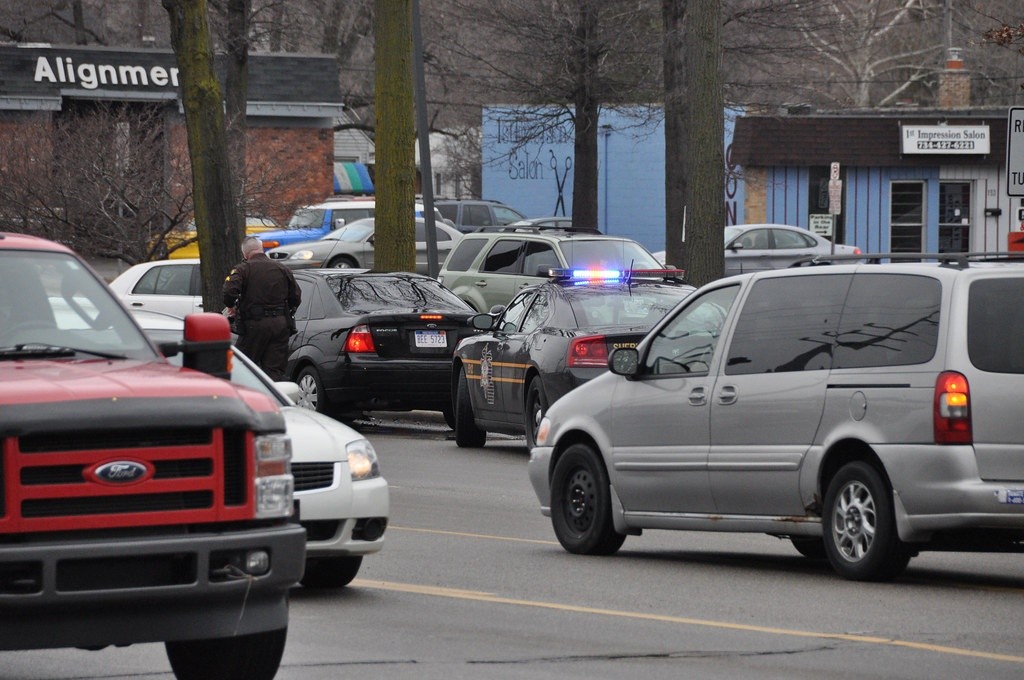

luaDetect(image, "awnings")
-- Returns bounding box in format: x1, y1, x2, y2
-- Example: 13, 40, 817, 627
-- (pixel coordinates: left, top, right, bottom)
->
334, 161, 375, 194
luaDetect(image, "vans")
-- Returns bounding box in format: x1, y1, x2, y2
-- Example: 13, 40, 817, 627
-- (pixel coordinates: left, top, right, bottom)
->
529, 250, 1023, 582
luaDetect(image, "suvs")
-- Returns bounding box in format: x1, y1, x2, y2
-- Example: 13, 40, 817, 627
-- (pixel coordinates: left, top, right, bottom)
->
436, 225, 667, 313
2, 232, 306, 679
254, 196, 459, 254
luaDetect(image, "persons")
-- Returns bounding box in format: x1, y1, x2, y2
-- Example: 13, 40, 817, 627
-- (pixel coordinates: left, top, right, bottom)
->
220, 236, 302, 383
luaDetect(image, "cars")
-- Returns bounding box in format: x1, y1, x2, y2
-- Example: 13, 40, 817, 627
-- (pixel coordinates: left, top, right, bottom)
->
422, 194, 531, 236
109, 257, 203, 319
288, 265, 485, 428
144, 214, 289, 261
450, 263, 730, 448
262, 216, 464, 282
51, 296, 393, 591
504, 216, 606, 236
653, 222, 863, 289
